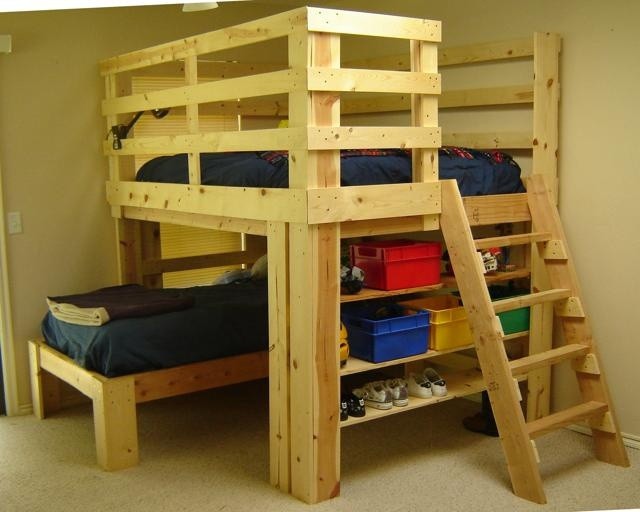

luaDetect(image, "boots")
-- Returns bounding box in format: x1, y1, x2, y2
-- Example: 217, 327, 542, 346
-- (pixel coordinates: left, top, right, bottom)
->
462, 390, 499, 438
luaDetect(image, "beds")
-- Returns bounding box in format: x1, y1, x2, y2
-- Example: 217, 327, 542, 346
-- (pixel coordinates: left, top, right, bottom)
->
19, 141, 529, 506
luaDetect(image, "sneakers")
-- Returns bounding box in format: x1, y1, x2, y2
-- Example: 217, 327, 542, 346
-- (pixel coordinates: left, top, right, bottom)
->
340, 367, 447, 421
